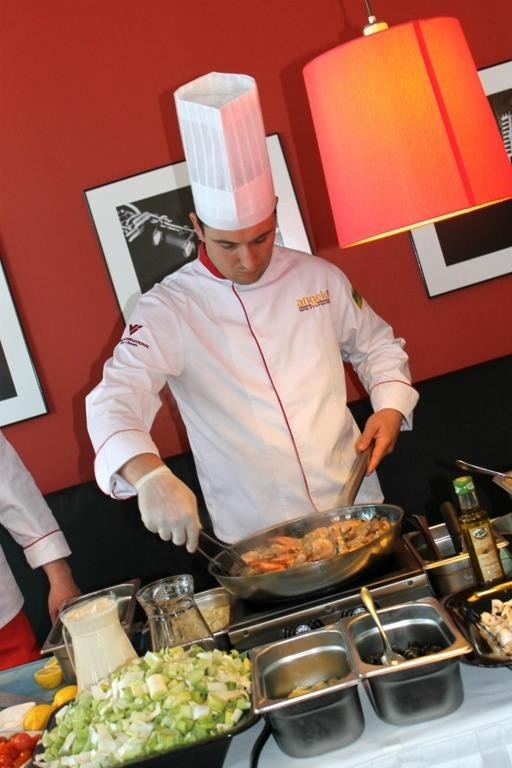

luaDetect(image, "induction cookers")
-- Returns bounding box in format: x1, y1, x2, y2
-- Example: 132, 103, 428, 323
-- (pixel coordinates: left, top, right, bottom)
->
227, 534, 437, 651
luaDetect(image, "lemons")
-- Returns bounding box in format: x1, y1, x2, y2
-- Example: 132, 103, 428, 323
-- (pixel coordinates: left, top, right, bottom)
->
24, 705, 54, 730
53, 685, 77, 707
35, 658, 61, 688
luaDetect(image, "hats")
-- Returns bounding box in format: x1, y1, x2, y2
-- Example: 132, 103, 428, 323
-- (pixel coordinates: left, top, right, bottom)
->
172, 71, 276, 232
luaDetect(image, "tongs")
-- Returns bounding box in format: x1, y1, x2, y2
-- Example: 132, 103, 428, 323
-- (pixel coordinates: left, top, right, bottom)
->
196, 529, 246, 577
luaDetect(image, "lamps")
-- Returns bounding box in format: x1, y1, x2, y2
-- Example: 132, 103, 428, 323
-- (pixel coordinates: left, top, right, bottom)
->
300, 0, 511, 249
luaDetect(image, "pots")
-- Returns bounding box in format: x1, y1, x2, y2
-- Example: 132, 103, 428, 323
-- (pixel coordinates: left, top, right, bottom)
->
207, 438, 403, 597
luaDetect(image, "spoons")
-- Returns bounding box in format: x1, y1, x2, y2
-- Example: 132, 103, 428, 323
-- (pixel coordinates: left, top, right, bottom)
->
359, 585, 407, 665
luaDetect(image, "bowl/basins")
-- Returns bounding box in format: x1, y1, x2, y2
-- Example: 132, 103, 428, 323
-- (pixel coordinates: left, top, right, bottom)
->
0, 698, 38, 732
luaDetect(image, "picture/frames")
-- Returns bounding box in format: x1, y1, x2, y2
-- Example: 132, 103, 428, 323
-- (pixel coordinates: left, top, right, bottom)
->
1, 258, 48, 430
83, 134, 317, 333
407, 58, 512, 296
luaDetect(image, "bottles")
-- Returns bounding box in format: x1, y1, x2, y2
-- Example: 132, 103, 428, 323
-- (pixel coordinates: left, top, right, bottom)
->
452, 475, 505, 582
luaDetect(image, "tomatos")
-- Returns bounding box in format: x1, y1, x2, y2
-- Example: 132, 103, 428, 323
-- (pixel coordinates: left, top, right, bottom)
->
0, 733, 41, 768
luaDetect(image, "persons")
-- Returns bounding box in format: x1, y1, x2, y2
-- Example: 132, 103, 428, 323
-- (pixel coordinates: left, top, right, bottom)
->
0, 430, 82, 672
86, 196, 419, 553
